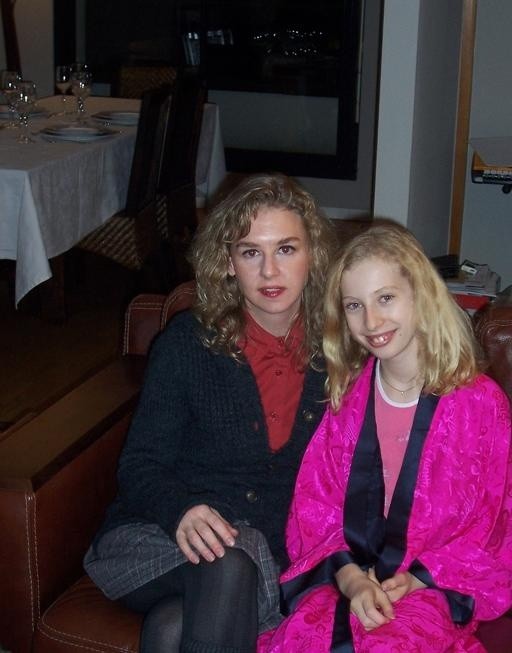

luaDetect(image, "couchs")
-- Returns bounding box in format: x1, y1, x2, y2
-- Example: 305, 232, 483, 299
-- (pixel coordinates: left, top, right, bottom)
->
0, 284, 512, 653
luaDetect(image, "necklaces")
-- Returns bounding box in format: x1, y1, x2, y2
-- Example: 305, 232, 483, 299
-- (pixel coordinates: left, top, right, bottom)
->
378, 364, 424, 400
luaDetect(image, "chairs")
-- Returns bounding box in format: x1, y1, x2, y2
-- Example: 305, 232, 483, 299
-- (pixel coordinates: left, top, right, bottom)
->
71, 64, 209, 306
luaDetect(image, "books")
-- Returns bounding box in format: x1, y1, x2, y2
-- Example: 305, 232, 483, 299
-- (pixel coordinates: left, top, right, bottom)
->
469, 135, 512, 185
428, 252, 503, 316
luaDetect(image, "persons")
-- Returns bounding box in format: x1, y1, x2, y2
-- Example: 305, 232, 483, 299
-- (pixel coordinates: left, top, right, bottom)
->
81, 172, 365, 653
252, 220, 512, 653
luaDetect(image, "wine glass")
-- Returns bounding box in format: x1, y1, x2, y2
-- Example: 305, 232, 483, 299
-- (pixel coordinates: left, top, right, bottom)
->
0, 70, 38, 144
55, 63, 93, 116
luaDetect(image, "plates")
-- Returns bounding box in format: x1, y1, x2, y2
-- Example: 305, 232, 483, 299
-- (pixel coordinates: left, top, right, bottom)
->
0, 104, 47, 118
93, 110, 140, 126
41, 124, 121, 142
